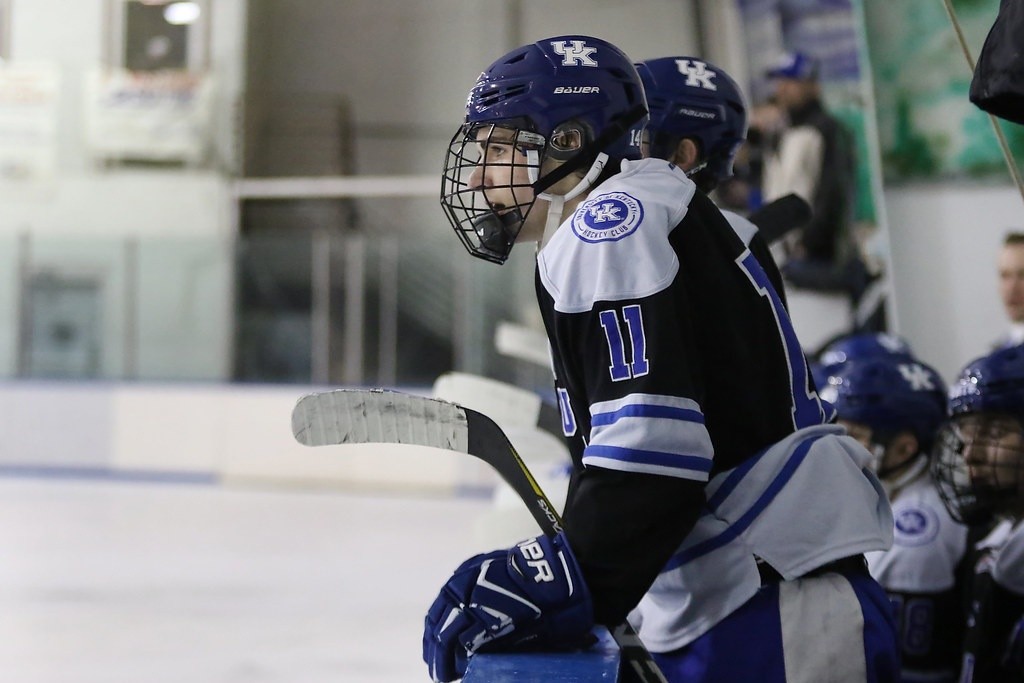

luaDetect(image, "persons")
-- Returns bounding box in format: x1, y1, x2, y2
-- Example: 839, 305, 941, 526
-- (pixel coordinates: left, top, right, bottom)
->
999, 233, 1024, 343
811, 329, 971, 683
939, 343, 1024, 683
422, 35, 898, 683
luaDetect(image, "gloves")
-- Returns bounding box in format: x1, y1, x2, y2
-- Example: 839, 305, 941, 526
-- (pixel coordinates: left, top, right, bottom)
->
423, 530, 598, 683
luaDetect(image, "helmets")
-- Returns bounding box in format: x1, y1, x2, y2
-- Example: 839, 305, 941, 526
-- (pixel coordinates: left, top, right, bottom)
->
929, 347, 1024, 524
819, 355, 947, 442
441, 36, 650, 265
809, 334, 911, 385
636, 56, 751, 183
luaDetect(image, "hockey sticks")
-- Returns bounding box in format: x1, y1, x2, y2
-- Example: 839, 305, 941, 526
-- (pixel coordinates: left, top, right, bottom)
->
492, 317, 555, 369
430, 368, 571, 448
284, 386, 673, 683
747, 192, 814, 248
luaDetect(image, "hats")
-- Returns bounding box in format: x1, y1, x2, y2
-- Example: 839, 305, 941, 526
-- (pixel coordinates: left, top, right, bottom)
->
765, 52, 821, 80
